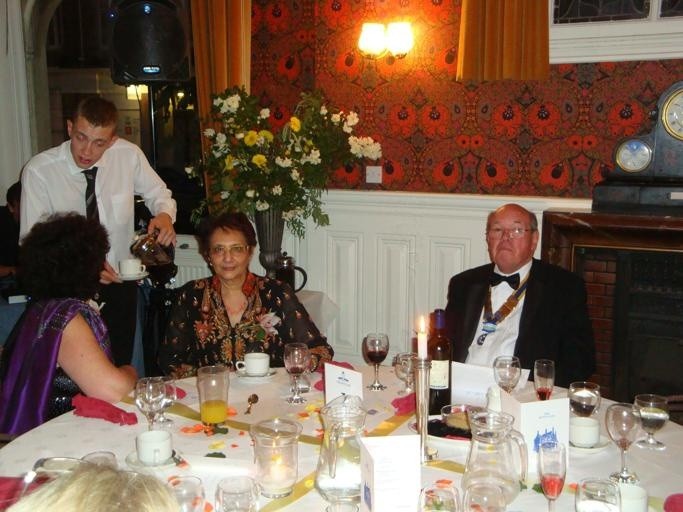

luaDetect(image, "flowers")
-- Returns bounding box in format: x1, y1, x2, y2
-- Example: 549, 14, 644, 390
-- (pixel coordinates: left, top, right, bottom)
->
185, 83, 383, 241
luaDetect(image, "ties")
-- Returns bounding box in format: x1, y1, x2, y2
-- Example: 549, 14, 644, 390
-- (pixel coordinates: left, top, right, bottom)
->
82, 167, 99, 224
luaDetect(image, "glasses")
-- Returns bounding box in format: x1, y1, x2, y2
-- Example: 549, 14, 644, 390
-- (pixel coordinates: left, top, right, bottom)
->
488, 227, 533, 239
212, 247, 244, 257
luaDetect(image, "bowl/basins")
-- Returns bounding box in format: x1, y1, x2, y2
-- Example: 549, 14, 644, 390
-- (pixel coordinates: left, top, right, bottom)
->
440, 403, 492, 431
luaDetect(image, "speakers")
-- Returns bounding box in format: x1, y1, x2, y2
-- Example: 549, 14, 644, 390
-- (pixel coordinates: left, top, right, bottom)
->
111, 0, 192, 85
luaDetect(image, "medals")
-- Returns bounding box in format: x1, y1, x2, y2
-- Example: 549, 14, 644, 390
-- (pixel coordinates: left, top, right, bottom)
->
477, 333, 486, 346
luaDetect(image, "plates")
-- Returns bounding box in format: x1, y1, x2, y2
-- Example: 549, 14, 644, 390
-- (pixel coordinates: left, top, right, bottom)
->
127, 453, 175, 470
118, 272, 149, 282
234, 369, 279, 381
409, 414, 472, 445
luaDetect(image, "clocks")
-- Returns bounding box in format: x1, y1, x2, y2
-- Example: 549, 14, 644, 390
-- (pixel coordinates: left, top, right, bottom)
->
616, 136, 652, 173
661, 86, 682, 142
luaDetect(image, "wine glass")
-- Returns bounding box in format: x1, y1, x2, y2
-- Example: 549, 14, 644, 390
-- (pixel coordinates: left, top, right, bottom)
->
606, 393, 670, 485
364, 333, 390, 390
394, 352, 417, 397
284, 343, 308, 405
538, 442, 567, 511
134, 376, 177, 433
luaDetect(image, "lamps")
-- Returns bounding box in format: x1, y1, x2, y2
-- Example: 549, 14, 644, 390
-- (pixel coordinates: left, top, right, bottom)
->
357, 19, 413, 58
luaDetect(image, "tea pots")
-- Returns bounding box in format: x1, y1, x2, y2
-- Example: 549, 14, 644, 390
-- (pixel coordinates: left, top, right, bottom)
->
316, 395, 367, 512
131, 233, 178, 287
277, 252, 308, 293
462, 405, 528, 504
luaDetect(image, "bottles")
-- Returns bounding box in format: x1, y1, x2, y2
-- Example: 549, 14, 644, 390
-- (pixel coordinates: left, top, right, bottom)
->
429, 309, 452, 413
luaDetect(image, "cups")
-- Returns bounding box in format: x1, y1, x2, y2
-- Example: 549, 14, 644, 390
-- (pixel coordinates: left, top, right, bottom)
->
235, 352, 271, 375
81, 450, 118, 469
119, 259, 146, 276
494, 356, 521, 394
567, 382, 601, 449
169, 419, 303, 512
134, 430, 173, 464
532, 360, 555, 400
574, 477, 648, 511
418, 483, 506, 512
197, 366, 231, 424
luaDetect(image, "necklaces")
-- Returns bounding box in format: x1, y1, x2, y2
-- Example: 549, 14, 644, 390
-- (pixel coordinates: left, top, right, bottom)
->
222, 295, 247, 316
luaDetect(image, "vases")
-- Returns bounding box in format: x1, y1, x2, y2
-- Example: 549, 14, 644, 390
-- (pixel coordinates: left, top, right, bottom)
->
255, 207, 285, 280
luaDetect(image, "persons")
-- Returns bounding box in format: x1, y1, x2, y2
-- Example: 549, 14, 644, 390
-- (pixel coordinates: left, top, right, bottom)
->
153, 212, 333, 379
18, 93, 179, 369
0, 211, 138, 449
1, 183, 20, 266
441, 206, 596, 391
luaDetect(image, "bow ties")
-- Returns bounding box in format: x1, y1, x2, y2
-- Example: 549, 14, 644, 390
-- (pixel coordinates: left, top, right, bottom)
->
490, 272, 520, 289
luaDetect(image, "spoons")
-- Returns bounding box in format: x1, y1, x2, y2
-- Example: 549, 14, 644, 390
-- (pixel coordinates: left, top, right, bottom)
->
245, 393, 258, 415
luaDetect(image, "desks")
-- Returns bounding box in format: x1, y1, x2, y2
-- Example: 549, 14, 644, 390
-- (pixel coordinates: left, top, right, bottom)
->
292, 290, 341, 335
